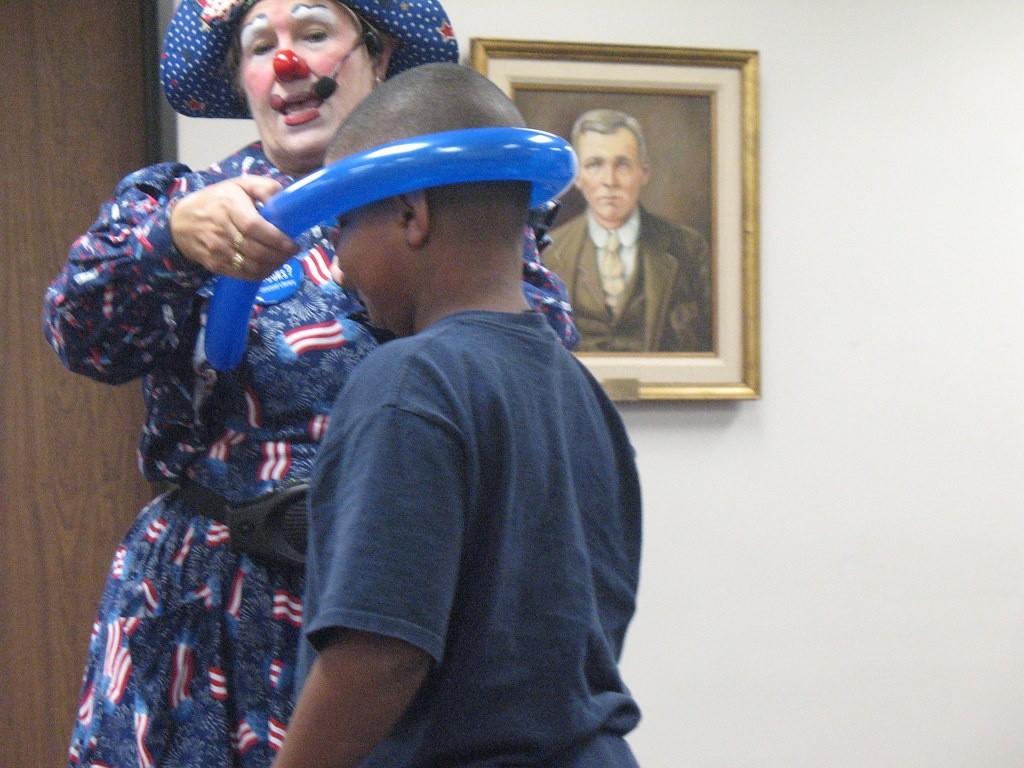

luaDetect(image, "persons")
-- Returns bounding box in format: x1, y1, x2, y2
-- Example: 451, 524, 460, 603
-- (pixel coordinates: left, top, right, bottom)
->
43, 0, 582, 768
270, 61, 642, 768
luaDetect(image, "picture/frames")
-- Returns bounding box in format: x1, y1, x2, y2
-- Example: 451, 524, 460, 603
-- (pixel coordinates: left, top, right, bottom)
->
470, 37, 761, 401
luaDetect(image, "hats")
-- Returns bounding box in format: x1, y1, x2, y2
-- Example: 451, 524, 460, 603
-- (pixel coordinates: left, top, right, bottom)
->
160, 0, 459, 119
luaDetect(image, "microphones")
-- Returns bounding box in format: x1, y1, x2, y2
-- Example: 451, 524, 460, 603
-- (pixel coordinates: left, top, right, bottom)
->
315, 36, 366, 100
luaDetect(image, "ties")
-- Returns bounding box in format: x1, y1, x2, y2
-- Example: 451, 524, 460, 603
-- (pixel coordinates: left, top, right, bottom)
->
599, 230, 632, 319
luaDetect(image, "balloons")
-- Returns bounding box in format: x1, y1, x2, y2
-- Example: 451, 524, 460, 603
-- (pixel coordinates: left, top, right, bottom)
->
204, 124, 579, 373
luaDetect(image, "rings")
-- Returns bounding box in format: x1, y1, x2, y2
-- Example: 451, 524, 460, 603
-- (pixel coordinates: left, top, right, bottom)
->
232, 253, 246, 273
234, 231, 244, 251
